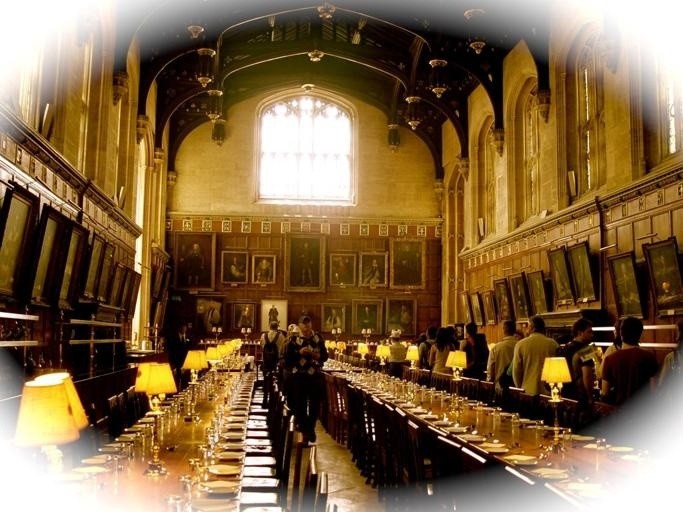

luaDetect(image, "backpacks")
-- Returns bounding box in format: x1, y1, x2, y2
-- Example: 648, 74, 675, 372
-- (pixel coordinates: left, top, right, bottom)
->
263, 331, 279, 367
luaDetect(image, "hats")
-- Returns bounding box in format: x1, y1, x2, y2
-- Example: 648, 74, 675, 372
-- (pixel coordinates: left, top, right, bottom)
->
389, 331, 399, 338
299, 316, 311, 323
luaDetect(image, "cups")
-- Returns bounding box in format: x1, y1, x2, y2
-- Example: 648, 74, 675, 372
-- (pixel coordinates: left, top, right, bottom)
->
321, 349, 655, 502
199, 337, 262, 345
345, 337, 416, 346
61, 354, 255, 509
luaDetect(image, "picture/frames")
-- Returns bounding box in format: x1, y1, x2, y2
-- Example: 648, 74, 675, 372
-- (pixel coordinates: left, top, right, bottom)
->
456, 235, 682, 326
0, 181, 142, 314
172, 230, 428, 337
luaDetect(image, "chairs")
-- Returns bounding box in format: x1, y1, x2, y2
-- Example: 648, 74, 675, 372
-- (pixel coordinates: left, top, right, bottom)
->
91, 362, 329, 512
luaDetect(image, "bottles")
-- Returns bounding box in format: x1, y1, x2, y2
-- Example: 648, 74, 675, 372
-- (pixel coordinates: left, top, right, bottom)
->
0, 319, 31, 342
26, 351, 54, 377
141, 335, 153, 350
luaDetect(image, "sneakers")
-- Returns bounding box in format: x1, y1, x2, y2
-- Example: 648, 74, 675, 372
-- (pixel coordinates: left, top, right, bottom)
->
302, 428, 316, 442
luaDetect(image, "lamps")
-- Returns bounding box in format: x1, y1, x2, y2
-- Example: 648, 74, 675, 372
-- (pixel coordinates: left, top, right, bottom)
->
180, 346, 222, 385
357, 343, 421, 369
16, 372, 92, 477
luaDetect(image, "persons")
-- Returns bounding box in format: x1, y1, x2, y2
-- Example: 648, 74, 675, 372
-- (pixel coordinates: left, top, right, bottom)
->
172, 322, 198, 367
655, 250, 675, 275
459, 322, 488, 378
515, 283, 524, 317
600, 316, 659, 407
255, 258, 273, 283
259, 314, 330, 444
576, 254, 586, 297
325, 308, 341, 328
178, 242, 205, 287
553, 260, 570, 299
388, 331, 406, 360
228, 256, 245, 281
487, 319, 519, 383
333, 256, 352, 284
267, 304, 279, 325
498, 329, 529, 389
598, 316, 626, 376
417, 325, 435, 368
511, 316, 559, 398
428, 327, 455, 375
296, 239, 315, 285
364, 257, 381, 285
557, 317, 596, 407
658, 317, 682, 403
446, 325, 460, 350
399, 303, 412, 332
358, 305, 374, 329
513, 329, 524, 341
237, 305, 250, 327
203, 301, 220, 330
